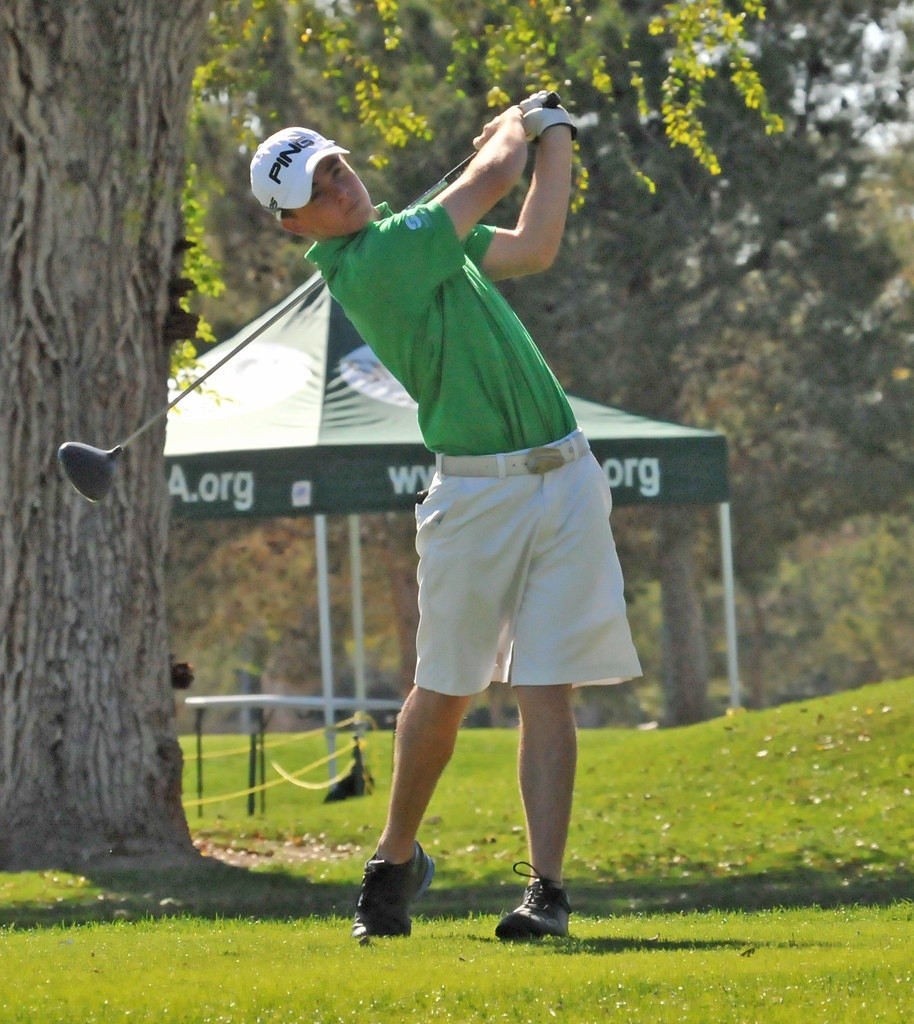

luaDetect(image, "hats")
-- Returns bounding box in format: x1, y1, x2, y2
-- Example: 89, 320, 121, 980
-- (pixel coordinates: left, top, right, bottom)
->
249, 127, 350, 222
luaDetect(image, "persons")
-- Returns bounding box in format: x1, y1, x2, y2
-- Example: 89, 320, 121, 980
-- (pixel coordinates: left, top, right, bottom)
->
250, 87, 643, 940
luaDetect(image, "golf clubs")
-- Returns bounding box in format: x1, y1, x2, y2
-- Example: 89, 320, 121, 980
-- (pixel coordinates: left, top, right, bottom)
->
56, 89, 561, 503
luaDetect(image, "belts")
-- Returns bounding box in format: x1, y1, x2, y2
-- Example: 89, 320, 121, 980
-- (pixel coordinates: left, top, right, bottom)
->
435, 428, 591, 477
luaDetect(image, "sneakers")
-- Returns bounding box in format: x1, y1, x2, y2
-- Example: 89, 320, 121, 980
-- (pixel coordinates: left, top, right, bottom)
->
350, 840, 434, 946
495, 862, 572, 939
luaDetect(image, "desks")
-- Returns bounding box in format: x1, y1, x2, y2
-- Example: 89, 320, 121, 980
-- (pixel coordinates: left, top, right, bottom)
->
187, 694, 400, 817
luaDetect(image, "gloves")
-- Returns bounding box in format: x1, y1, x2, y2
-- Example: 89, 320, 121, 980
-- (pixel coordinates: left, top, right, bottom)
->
519, 89, 578, 143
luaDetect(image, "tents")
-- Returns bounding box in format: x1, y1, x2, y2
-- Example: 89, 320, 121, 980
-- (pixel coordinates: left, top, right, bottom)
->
166, 267, 741, 796
